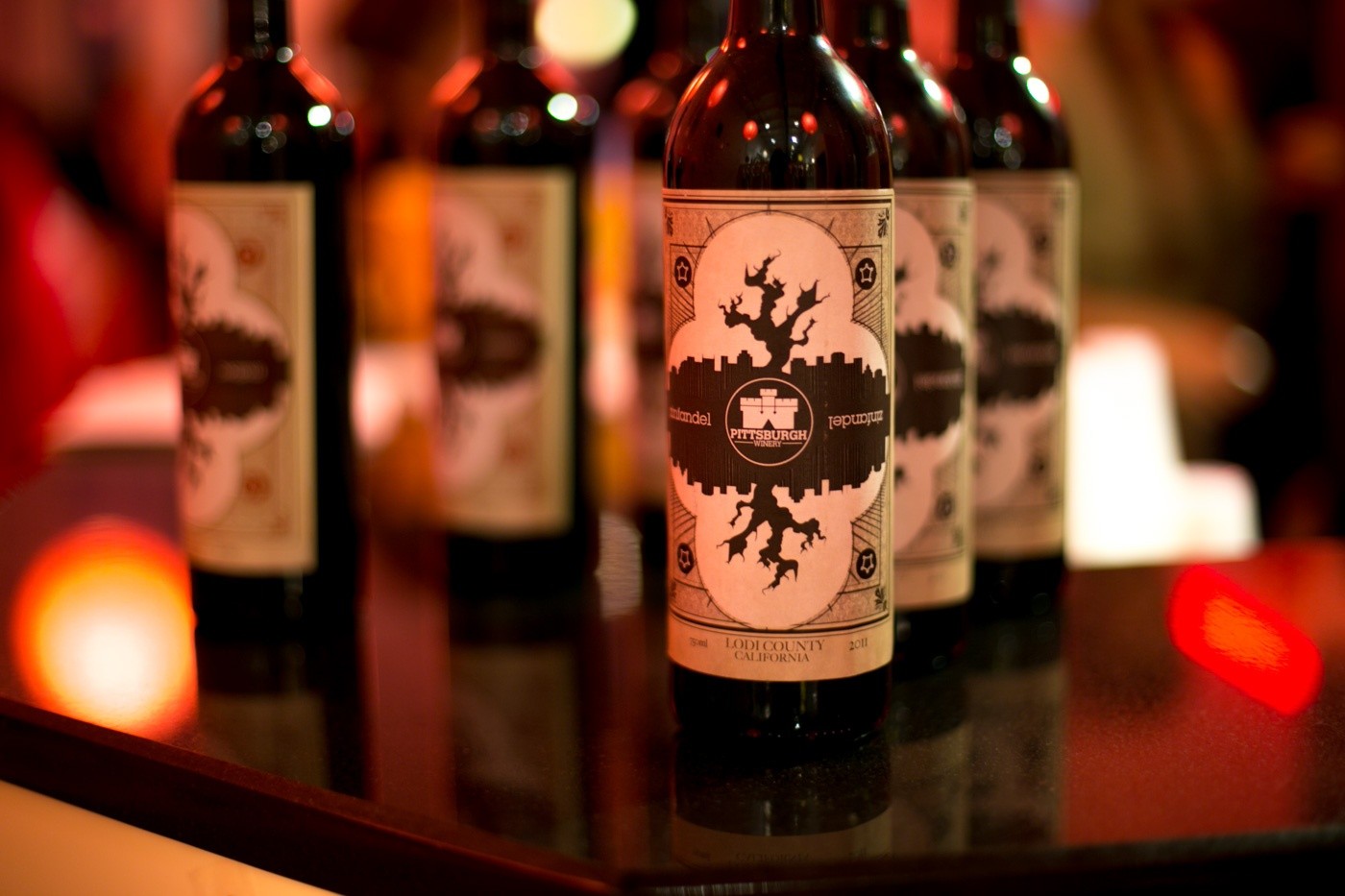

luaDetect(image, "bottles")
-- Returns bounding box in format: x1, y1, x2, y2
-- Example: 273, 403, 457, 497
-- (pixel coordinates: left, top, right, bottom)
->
162, 1, 1081, 835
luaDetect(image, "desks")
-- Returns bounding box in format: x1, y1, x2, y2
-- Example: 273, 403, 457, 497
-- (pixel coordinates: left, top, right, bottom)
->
0, 453, 1345, 896
